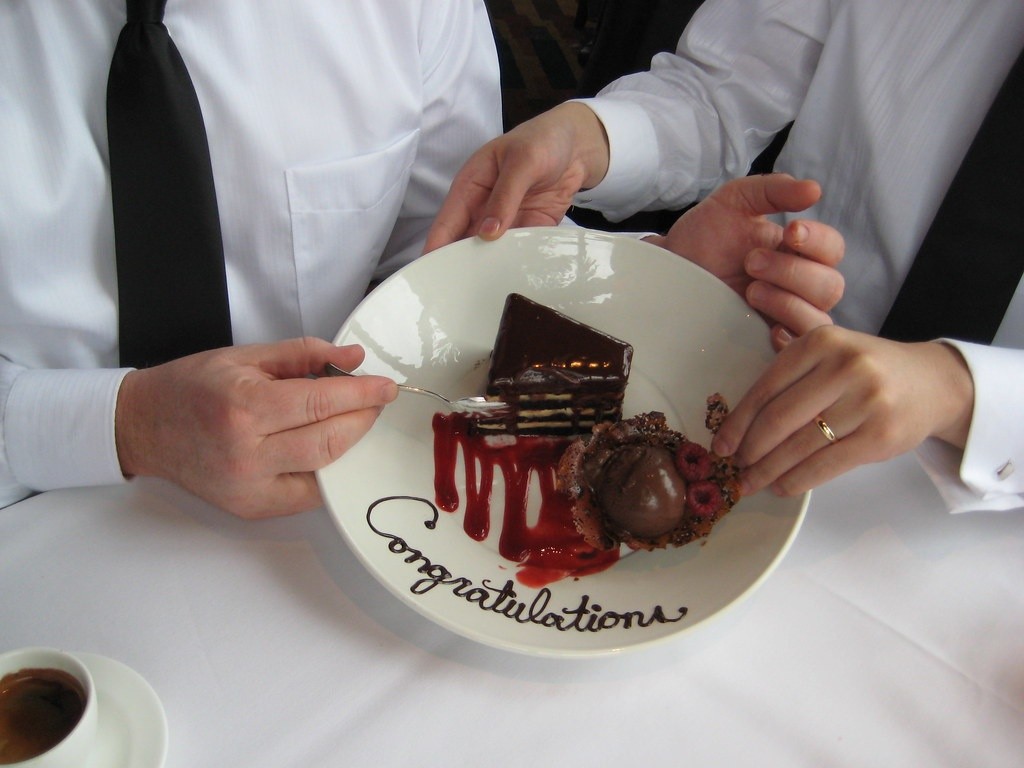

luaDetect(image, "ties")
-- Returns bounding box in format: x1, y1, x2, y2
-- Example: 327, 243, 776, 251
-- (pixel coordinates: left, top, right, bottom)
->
878, 51, 1023, 345
105, 1, 233, 368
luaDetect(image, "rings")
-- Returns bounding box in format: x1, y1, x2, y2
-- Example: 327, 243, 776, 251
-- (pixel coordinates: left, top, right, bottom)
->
815, 417, 839, 444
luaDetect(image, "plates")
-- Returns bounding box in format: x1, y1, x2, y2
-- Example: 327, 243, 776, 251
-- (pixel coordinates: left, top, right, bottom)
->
62, 651, 168, 768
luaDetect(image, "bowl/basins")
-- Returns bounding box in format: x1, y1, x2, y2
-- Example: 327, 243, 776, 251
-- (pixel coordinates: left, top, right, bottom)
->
312, 228, 811, 659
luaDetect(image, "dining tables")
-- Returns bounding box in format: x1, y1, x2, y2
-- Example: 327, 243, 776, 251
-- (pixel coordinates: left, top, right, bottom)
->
0, 433, 1024, 768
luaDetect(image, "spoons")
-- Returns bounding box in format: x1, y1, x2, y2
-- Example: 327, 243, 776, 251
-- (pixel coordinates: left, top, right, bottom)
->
323, 359, 518, 421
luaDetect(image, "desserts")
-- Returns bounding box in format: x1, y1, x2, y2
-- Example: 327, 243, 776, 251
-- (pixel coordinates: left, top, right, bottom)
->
476, 292, 633, 436
558, 391, 737, 553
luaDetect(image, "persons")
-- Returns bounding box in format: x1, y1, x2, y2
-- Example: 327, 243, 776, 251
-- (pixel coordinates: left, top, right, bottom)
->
424, 0, 1024, 512
0, 0, 845, 519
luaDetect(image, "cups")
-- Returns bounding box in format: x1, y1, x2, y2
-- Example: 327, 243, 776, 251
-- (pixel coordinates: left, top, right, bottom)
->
0, 647, 98, 766
